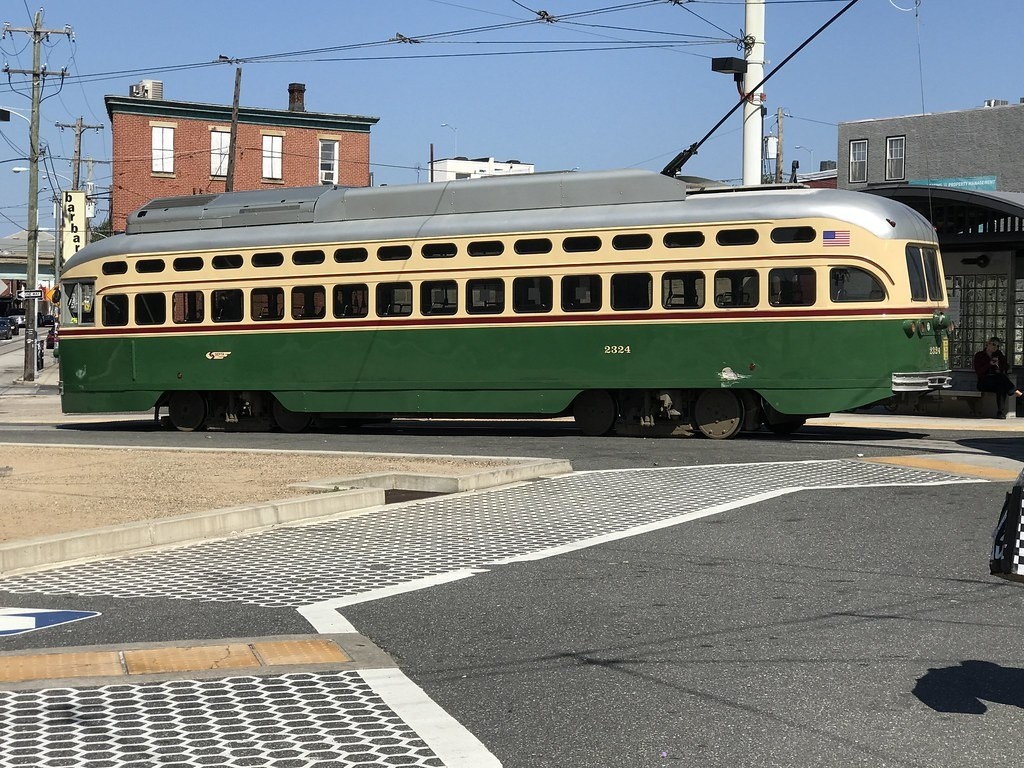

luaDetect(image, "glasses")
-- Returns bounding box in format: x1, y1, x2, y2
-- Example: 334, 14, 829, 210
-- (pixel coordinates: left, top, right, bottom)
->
991, 343, 1000, 348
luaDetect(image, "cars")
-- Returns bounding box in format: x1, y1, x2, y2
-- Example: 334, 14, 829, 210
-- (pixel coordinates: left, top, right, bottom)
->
8, 308, 27, 327
38, 312, 56, 327
0, 317, 20, 340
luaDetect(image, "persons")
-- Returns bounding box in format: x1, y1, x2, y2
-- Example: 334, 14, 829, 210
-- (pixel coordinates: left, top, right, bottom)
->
741, 277, 758, 305
974, 337, 1024, 419
217, 293, 235, 318
318, 289, 342, 316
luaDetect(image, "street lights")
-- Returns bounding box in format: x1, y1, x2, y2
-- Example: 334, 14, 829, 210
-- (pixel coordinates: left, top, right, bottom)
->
12, 167, 80, 191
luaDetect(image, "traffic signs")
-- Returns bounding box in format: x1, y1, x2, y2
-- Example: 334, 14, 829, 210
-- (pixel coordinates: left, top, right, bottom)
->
16, 289, 45, 301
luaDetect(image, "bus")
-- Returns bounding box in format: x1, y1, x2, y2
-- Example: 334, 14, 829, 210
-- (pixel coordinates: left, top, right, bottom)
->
52, 168, 957, 440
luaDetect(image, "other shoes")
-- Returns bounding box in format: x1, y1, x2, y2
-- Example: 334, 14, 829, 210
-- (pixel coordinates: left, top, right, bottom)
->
1016, 393, 1024, 399
997, 412, 1005, 419
